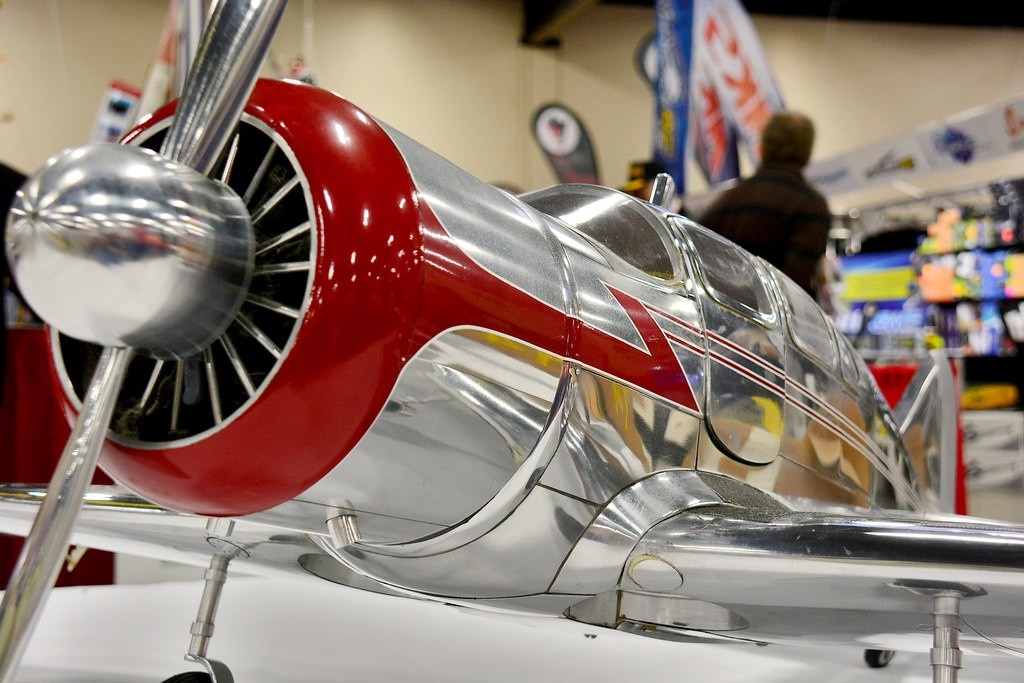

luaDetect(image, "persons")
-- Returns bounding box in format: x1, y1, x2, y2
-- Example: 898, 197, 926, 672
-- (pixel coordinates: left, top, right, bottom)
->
698, 112, 834, 306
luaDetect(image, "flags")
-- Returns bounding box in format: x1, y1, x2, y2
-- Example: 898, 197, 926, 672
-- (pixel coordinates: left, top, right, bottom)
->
656, 1, 789, 224
126, 1, 205, 135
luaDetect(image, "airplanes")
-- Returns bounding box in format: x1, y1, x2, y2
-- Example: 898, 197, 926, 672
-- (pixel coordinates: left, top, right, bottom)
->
0, 0, 1024, 682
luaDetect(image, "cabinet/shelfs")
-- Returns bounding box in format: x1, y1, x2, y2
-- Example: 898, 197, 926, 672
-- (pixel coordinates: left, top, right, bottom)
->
841, 179, 1022, 492
